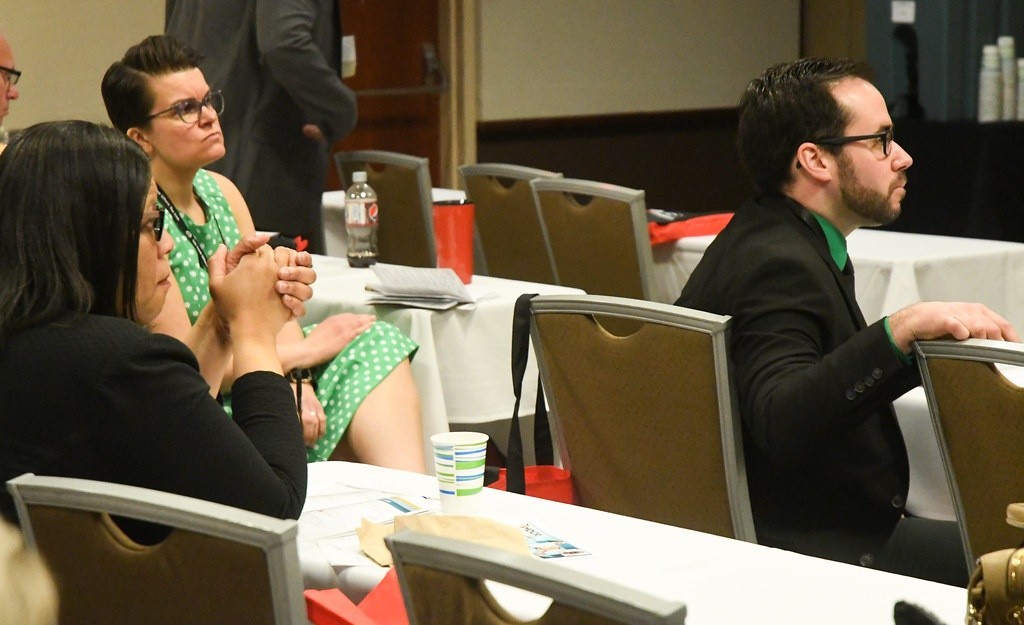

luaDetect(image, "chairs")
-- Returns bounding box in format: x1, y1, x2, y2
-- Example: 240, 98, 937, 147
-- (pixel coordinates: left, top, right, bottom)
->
383, 527, 687, 625
456, 164, 562, 288
334, 149, 437, 269
6, 473, 308, 625
529, 178, 661, 305
517, 295, 756, 546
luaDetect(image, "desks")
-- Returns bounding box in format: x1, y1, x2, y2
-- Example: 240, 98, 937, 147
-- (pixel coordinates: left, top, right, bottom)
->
296, 461, 968, 625
297, 253, 585, 483
895, 118, 1024, 244
322, 185, 1024, 342
891, 338, 1024, 579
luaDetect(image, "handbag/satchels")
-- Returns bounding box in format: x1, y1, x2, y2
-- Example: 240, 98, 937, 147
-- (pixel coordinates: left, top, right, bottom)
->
458, 291, 575, 504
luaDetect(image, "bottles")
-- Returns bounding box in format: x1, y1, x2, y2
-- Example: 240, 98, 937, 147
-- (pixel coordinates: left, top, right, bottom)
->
344, 171, 379, 270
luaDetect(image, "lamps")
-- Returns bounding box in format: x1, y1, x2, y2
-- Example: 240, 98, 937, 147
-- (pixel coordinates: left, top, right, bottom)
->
888, 0, 929, 122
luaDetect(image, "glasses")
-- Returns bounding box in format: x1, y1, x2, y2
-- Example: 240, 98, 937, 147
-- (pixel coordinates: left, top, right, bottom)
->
146, 89, 225, 124
137, 206, 167, 241
0, 64, 23, 86
795, 127, 895, 170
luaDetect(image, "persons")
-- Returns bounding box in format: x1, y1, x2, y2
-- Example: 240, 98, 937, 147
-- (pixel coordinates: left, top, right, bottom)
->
164, 0, 358, 252
0, 120, 317, 545
0, 33, 22, 124
672, 57, 1024, 587
101, 34, 425, 473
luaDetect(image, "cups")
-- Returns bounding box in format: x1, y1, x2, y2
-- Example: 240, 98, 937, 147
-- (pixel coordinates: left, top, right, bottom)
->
430, 432, 489, 514
431, 198, 475, 285
977, 35, 1024, 123
